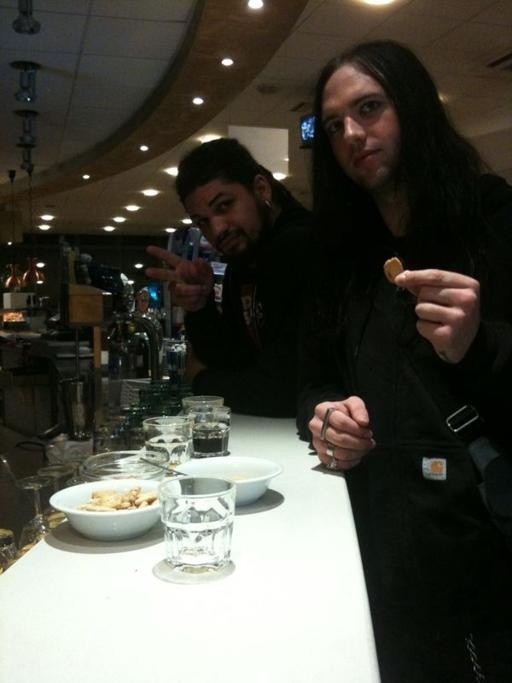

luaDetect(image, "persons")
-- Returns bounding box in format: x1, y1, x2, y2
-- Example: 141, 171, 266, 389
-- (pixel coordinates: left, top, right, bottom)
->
294, 35, 512, 683
127, 290, 163, 379
142, 137, 317, 419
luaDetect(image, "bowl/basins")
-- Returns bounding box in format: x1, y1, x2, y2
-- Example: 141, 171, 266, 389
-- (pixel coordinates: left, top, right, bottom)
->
173, 456, 284, 508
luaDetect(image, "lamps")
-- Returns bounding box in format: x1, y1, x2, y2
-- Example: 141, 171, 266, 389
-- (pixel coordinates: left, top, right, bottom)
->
16, 116, 37, 147
9, 60, 42, 115
3, 170, 25, 289
21, 149, 32, 169
11, 0, 41, 34
23, 164, 48, 286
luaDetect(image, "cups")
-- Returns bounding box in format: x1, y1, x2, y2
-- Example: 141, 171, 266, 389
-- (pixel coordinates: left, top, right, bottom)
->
142, 394, 238, 578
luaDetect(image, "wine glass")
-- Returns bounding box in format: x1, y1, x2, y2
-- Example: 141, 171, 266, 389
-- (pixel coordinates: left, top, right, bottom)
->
0, 398, 154, 575
166, 226, 208, 313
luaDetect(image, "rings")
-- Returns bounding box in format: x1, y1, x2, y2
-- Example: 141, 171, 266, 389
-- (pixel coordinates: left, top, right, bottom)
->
325, 442, 338, 458
319, 407, 341, 442
325, 456, 336, 472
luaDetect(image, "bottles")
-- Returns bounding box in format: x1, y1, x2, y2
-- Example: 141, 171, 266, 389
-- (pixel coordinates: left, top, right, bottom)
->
146, 306, 188, 370
